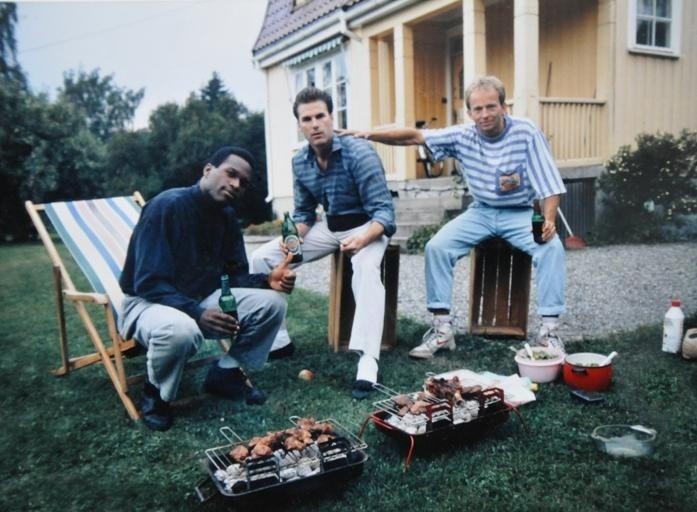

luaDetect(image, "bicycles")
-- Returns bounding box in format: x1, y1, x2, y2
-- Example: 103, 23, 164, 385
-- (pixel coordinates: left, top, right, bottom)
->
415, 117, 443, 178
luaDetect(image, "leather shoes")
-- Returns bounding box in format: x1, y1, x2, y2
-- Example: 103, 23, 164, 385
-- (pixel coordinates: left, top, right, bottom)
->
268, 343, 295, 361
350, 379, 374, 401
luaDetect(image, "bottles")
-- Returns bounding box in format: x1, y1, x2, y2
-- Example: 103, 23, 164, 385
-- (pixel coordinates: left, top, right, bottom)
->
283, 211, 303, 263
532, 199, 546, 245
218, 276, 237, 321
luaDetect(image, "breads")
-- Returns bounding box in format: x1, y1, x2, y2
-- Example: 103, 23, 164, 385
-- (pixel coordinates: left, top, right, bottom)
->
298, 369, 313, 381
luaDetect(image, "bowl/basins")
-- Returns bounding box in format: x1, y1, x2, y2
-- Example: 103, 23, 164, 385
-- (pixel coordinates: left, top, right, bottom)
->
592, 424, 658, 458
514, 347, 564, 383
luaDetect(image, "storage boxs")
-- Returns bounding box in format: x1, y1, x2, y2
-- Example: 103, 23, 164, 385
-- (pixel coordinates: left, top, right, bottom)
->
328, 242, 399, 354
466, 235, 532, 341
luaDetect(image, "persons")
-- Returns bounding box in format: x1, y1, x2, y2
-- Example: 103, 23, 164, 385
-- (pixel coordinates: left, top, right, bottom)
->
116, 145, 296, 431
335, 75, 568, 360
250, 85, 397, 401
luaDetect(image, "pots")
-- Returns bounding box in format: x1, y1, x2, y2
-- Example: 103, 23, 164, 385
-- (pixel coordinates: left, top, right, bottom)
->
564, 352, 612, 392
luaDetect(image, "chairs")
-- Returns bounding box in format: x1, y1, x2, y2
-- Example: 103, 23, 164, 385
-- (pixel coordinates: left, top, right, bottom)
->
24, 189, 257, 425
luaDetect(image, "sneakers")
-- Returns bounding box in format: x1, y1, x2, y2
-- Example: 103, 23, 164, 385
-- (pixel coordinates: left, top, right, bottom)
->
139, 387, 171, 431
409, 317, 457, 359
203, 360, 265, 405
537, 322, 565, 354
